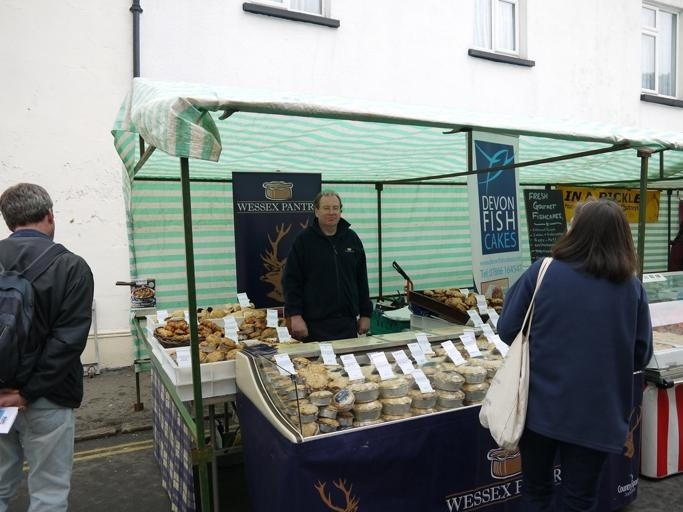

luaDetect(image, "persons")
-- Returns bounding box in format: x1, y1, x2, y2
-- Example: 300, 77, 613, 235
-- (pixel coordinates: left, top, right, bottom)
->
0, 182, 93, 512
497, 196, 653, 511
277, 189, 372, 344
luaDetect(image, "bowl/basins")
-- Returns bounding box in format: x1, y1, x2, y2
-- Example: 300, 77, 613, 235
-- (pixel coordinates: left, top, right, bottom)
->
261, 337, 506, 436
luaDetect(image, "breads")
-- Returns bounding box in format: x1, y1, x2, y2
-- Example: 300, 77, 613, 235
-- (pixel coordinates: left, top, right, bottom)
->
424, 288, 503, 313
262, 339, 504, 435
157, 302, 286, 364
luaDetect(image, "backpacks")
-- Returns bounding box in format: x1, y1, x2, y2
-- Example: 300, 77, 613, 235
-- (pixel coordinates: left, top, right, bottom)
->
0, 244, 68, 389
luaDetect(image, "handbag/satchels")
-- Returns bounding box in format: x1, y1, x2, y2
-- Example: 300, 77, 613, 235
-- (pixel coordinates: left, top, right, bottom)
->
478, 333, 530, 452
